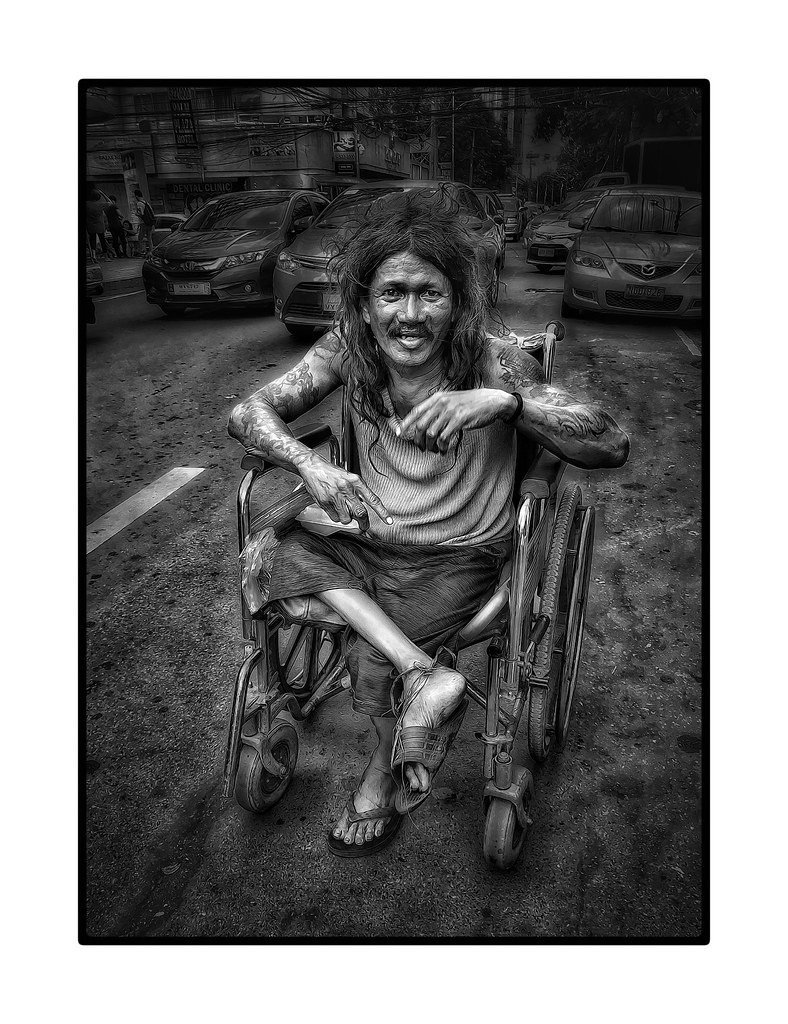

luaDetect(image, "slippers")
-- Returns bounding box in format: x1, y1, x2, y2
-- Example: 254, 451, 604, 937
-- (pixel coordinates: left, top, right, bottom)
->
391, 699, 469, 814
327, 788, 404, 858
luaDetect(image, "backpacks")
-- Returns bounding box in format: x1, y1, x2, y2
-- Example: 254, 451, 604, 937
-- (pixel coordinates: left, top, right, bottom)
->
86, 263, 105, 297
136, 201, 156, 225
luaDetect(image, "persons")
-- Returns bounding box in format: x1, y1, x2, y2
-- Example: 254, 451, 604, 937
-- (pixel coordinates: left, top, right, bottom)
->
224, 198, 629, 858
85, 188, 157, 263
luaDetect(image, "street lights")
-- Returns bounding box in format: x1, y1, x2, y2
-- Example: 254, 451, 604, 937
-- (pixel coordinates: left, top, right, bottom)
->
452, 98, 479, 182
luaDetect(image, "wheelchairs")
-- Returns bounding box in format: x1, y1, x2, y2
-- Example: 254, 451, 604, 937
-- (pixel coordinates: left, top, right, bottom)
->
221, 322, 596, 869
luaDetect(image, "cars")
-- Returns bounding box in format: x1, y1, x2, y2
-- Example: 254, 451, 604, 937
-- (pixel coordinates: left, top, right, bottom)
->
472, 188, 545, 248
562, 187, 702, 319
142, 189, 332, 317
523, 185, 688, 272
272, 179, 505, 335
152, 214, 189, 247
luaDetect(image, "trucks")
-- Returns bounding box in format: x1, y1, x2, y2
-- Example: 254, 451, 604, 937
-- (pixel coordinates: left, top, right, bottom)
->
580, 136, 702, 192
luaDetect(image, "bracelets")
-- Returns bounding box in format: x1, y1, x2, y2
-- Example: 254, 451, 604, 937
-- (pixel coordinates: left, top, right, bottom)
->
503, 393, 523, 425
